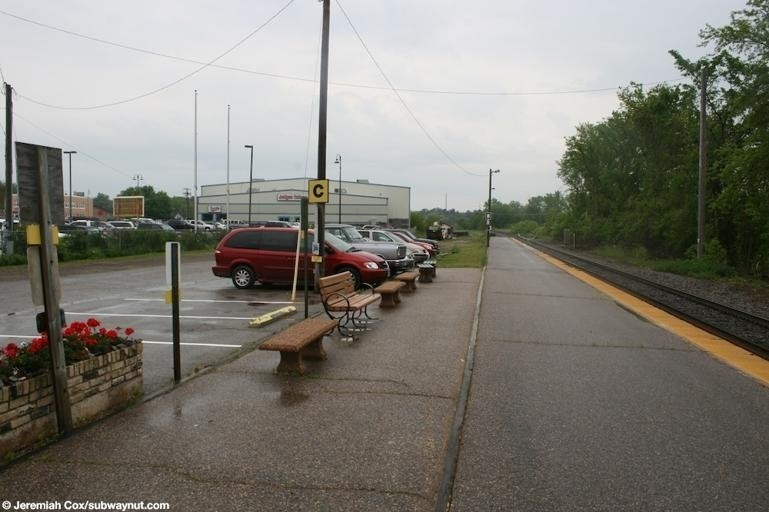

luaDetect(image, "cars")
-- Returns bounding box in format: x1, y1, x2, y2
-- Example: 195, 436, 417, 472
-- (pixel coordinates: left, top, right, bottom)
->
0, 218, 300, 239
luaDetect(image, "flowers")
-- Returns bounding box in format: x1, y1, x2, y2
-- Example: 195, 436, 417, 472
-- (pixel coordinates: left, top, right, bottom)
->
0, 316, 135, 374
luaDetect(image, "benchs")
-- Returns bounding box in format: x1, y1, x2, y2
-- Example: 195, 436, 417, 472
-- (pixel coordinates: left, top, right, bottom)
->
424, 260, 437, 277
319, 270, 381, 343
394, 272, 421, 294
374, 281, 407, 309
257, 317, 340, 375
418, 264, 433, 283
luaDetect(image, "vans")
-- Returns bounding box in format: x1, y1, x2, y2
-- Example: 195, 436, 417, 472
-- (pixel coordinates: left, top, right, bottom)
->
212, 227, 390, 291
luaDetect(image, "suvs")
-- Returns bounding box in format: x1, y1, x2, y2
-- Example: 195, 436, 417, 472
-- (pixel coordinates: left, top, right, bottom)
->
315, 223, 410, 281
352, 221, 441, 264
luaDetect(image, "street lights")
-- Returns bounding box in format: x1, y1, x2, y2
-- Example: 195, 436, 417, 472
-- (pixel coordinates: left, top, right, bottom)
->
63, 150, 77, 223
333, 153, 342, 224
486, 167, 500, 247
133, 173, 143, 195
244, 143, 254, 225
